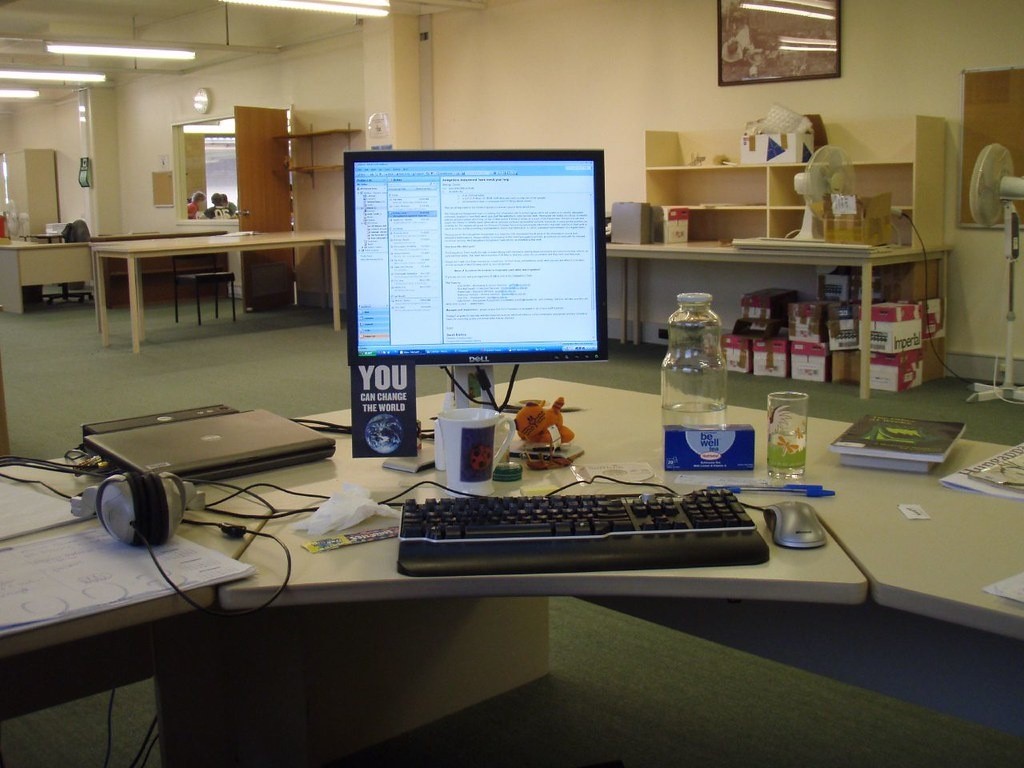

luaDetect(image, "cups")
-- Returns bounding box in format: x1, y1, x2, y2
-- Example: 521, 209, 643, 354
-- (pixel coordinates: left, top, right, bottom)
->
767, 392, 809, 480
437, 408, 516, 498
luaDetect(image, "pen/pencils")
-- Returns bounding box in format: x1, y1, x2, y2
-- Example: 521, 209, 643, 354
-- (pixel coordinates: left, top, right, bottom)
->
707, 484, 835, 498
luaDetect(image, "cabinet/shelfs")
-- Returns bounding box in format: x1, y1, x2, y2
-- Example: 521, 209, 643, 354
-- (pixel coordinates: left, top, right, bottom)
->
274, 129, 362, 172
91, 231, 227, 309
642, 116, 944, 249
3, 149, 58, 241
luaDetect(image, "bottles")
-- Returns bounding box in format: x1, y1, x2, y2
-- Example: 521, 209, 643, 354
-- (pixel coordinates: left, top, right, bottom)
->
660, 293, 727, 425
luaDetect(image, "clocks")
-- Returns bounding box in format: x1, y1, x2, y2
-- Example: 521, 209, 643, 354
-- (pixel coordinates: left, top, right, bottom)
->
194, 89, 210, 114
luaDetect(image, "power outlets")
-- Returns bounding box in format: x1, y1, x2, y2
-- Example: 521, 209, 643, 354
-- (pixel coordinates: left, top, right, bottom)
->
892, 206, 912, 225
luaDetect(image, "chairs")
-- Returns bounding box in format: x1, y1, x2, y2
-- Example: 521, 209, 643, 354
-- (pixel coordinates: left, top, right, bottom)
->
38, 220, 95, 306
173, 254, 236, 325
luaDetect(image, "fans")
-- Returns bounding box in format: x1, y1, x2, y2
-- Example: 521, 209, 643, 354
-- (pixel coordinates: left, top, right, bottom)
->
966, 144, 1024, 403
793, 145, 856, 243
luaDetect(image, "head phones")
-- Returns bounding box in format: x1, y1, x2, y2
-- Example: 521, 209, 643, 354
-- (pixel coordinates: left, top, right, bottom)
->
70, 471, 206, 546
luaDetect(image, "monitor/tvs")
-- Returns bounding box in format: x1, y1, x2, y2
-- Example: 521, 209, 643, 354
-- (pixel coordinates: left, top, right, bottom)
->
343, 149, 608, 474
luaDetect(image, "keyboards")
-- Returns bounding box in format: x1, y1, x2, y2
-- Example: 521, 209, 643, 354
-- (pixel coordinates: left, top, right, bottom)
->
396, 490, 771, 577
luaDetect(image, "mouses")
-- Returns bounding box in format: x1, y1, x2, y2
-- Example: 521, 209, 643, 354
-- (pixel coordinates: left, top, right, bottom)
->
764, 501, 828, 550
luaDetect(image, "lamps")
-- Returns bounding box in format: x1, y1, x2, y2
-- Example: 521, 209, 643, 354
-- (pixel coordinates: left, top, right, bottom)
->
0, 41, 195, 97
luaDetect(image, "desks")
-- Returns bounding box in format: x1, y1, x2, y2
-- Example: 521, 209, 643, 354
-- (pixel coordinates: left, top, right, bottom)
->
0, 379, 1024, 768
606, 244, 955, 398
0, 235, 345, 352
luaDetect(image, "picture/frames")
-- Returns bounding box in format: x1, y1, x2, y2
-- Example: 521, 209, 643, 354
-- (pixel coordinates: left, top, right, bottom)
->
956, 66, 1024, 228
717, 0, 841, 87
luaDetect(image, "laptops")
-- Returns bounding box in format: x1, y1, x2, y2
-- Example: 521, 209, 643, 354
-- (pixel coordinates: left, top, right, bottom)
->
81, 404, 339, 488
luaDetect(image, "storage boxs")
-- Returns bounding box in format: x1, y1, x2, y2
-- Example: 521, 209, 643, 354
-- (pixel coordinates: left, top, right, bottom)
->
610, 201, 690, 245
721, 270, 943, 392
742, 135, 814, 164
664, 425, 756, 470
